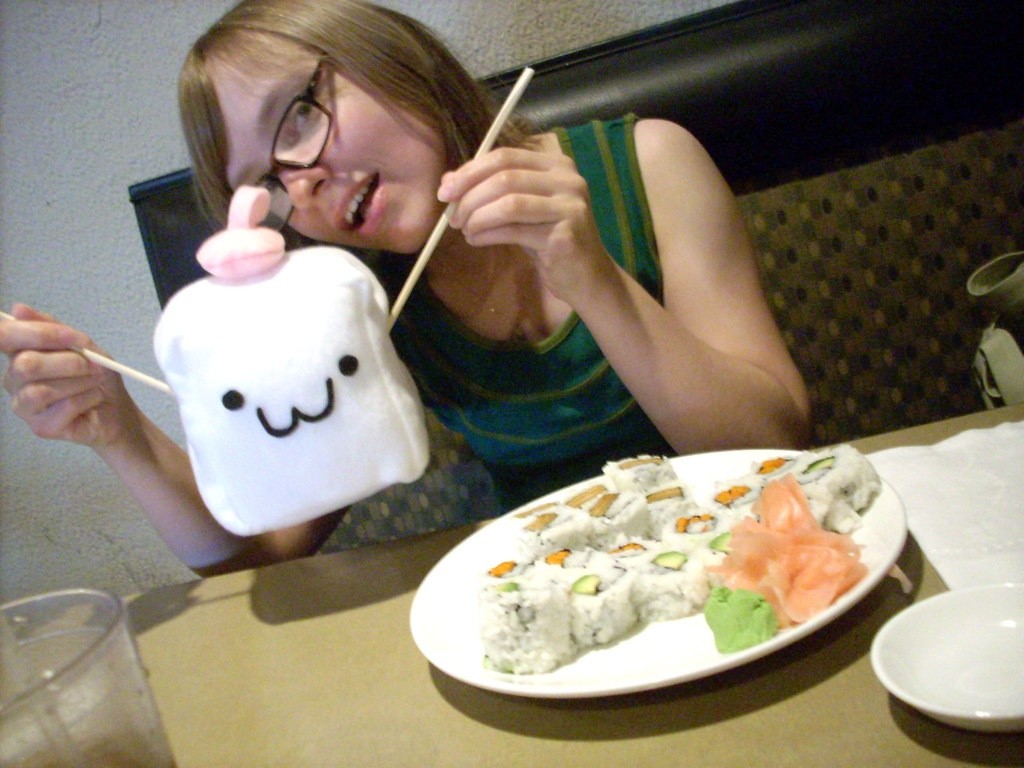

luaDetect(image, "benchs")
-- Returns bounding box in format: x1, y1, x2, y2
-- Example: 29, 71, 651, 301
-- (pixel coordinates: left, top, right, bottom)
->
125, 0, 1024, 554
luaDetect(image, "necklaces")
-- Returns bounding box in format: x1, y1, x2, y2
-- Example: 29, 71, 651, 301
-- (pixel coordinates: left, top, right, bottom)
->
433, 254, 523, 336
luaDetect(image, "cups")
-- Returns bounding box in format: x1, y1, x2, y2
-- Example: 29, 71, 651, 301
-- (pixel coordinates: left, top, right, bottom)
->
0, 583, 180, 768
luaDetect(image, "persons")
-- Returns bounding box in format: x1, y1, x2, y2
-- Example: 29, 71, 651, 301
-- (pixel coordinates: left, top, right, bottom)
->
1, 1, 814, 578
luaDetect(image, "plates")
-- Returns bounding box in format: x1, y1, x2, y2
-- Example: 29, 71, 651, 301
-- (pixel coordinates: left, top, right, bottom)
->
409, 448, 907, 700
870, 583, 1024, 732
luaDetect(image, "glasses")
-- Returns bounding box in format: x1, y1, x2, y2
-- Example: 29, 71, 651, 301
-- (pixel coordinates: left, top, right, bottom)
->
245, 96, 331, 228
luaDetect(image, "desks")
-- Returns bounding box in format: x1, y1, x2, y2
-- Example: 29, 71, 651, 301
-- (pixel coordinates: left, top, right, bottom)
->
0, 402, 1024, 768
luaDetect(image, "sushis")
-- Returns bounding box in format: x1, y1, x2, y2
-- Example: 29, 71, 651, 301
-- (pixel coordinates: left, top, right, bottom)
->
477, 443, 885, 674
